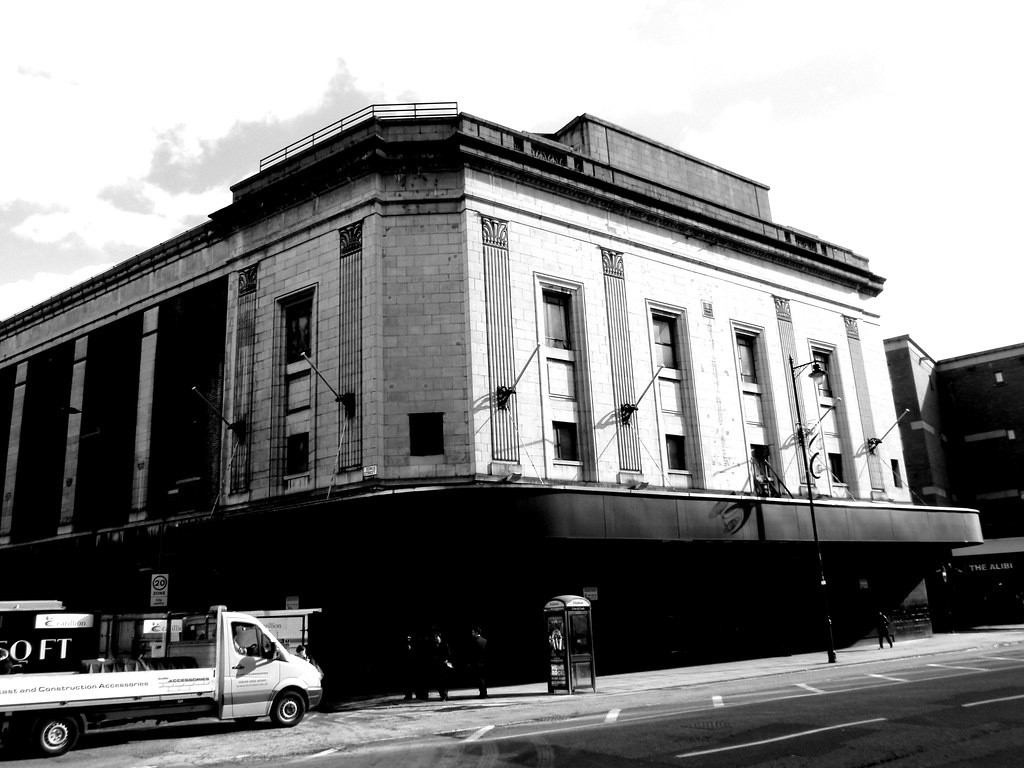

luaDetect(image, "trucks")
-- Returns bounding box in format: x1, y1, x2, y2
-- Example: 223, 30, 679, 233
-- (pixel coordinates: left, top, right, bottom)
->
0, 605, 324, 758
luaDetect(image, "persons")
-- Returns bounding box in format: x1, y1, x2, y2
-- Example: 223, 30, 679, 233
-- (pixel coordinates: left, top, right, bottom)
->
232, 630, 248, 656
434, 631, 454, 702
876, 611, 894, 649
294, 644, 316, 665
467, 627, 491, 699
400, 631, 424, 700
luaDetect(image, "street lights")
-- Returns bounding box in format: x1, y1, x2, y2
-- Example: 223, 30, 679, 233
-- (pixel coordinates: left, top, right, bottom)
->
788, 359, 840, 665
